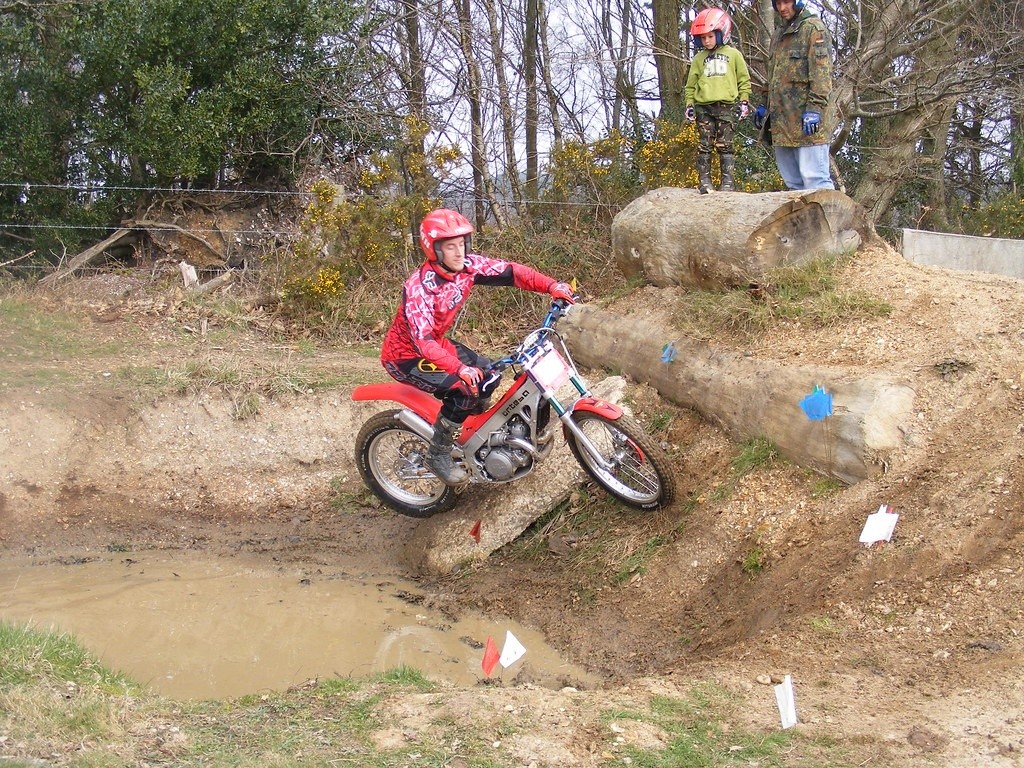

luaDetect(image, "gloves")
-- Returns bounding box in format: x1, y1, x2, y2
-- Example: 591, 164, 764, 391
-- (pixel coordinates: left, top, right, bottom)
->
456, 364, 485, 387
802, 112, 820, 136
738, 101, 749, 121
684, 107, 695, 121
548, 282, 576, 305
755, 104, 766, 130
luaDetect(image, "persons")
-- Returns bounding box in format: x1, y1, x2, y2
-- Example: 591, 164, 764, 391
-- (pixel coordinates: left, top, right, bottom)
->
753, 0, 837, 191
685, 8, 753, 194
381, 208, 575, 486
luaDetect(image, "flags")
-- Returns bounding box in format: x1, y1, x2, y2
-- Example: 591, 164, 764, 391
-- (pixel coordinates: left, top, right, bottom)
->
499, 630, 526, 669
798, 384, 833, 420
775, 675, 798, 729
481, 636, 500, 676
858, 505, 899, 546
660, 342, 678, 367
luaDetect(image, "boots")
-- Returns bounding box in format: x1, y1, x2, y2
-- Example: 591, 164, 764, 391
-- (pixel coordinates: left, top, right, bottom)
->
718, 152, 736, 192
696, 152, 714, 195
423, 411, 469, 485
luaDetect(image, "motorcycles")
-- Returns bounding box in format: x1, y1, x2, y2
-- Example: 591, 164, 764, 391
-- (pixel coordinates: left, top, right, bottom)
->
352, 276, 676, 518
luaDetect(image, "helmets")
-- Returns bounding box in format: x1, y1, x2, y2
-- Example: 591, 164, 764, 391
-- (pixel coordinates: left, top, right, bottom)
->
771, 0, 808, 13
419, 209, 474, 262
690, 8, 731, 48
